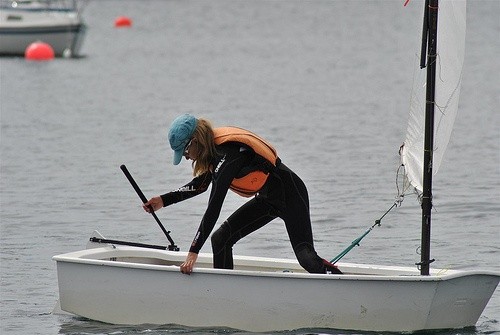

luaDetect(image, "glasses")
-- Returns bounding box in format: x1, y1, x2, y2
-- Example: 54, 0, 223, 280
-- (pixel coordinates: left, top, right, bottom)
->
183, 139, 192, 156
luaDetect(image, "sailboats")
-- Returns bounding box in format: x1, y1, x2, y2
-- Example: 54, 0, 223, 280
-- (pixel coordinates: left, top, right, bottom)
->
52, 0, 500, 333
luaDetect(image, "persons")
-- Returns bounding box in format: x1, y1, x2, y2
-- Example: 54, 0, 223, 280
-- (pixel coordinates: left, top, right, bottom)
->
143, 113, 344, 275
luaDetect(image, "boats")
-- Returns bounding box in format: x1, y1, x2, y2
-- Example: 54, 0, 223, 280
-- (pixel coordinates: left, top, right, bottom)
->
0, 0, 88, 57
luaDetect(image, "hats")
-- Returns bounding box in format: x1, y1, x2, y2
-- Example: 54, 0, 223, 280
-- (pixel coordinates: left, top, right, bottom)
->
167, 113, 198, 166
